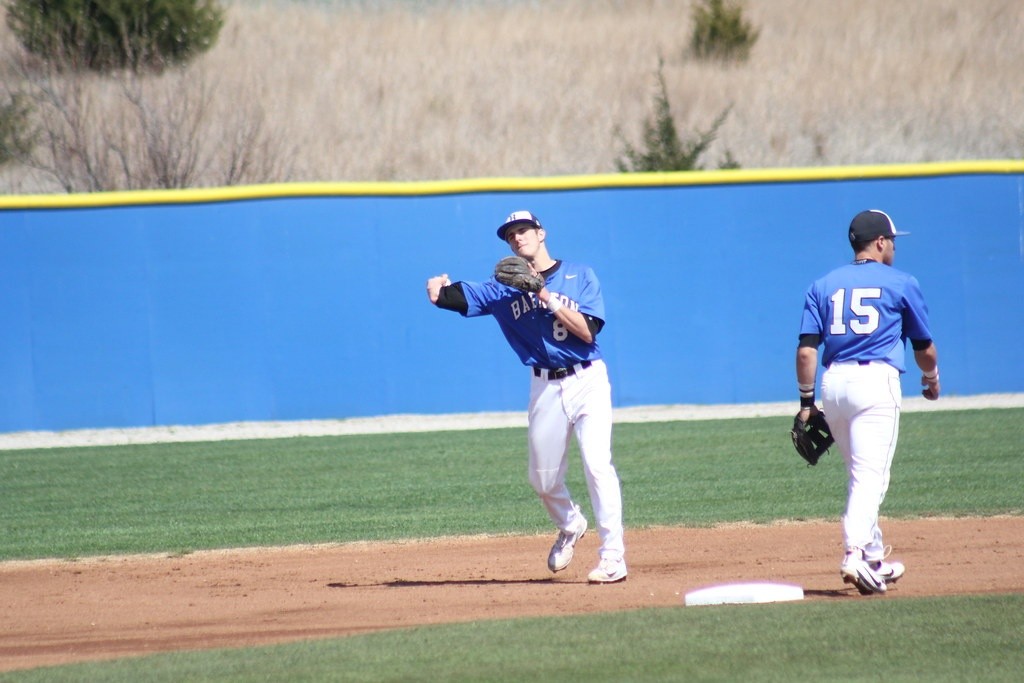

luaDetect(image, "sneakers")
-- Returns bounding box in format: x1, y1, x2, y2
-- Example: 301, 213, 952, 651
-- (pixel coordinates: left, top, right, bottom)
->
840, 546, 887, 594
587, 558, 627, 583
547, 512, 587, 572
869, 560, 905, 580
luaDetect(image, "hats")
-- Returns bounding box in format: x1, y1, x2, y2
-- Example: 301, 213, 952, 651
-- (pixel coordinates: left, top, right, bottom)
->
848, 209, 909, 240
497, 211, 543, 244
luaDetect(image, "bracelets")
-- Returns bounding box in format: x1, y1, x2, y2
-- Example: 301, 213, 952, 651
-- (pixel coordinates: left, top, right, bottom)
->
546, 294, 562, 312
427, 209, 629, 585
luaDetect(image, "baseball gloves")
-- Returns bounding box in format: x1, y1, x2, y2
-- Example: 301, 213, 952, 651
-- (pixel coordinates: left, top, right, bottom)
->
790, 408, 835, 466
494, 255, 545, 292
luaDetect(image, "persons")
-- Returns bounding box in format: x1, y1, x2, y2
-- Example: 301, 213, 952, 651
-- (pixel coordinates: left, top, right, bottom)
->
794, 210, 940, 597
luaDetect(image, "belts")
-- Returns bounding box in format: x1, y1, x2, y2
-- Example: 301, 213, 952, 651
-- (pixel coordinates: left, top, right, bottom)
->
534, 360, 592, 380
827, 360, 870, 366
